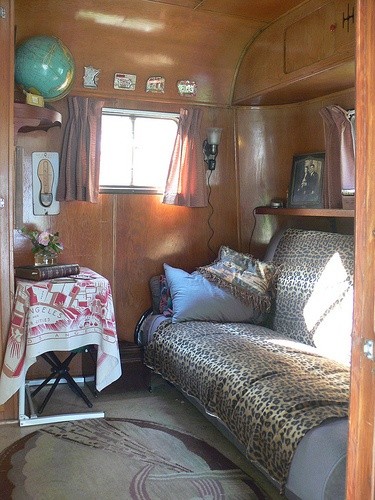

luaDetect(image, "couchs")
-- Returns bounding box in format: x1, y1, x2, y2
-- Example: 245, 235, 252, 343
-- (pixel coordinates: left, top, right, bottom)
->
134, 226, 355, 500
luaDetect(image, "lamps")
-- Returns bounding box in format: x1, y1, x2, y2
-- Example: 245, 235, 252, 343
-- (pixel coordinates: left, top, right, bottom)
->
202, 127, 225, 170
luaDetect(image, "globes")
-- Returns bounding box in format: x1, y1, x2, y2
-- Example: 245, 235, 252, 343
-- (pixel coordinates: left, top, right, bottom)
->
14, 33, 76, 103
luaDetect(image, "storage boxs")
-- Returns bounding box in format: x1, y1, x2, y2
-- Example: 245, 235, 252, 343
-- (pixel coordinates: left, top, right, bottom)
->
82, 340, 152, 399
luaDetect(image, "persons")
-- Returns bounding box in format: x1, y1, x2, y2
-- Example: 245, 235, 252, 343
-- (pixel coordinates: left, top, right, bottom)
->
297, 166, 309, 192
304, 164, 318, 195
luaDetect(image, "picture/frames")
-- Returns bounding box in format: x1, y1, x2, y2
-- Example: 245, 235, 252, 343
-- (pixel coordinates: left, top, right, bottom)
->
288, 152, 325, 208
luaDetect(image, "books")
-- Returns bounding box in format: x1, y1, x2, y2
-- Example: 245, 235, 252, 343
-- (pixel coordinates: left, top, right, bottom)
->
14, 263, 80, 281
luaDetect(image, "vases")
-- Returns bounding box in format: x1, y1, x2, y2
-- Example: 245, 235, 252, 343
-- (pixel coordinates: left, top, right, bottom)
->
35, 253, 53, 266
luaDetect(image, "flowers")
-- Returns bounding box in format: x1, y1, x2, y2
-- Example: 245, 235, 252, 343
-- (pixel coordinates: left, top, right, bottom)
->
15, 225, 64, 257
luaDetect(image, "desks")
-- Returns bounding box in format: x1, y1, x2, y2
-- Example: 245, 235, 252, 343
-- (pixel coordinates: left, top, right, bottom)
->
0, 267, 122, 414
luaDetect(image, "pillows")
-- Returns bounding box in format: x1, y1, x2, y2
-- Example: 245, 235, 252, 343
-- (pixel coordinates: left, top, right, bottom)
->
163, 263, 268, 328
160, 276, 173, 317
199, 244, 275, 313
